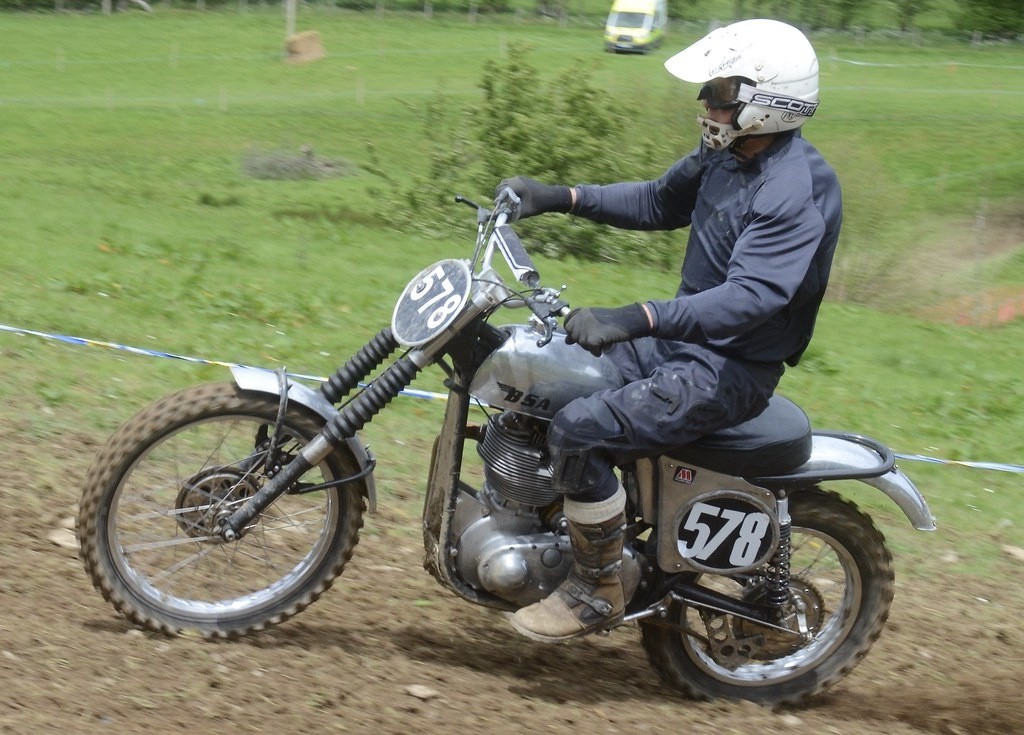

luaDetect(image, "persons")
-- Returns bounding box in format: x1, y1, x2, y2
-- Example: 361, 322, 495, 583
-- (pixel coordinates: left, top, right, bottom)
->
493, 18, 843, 644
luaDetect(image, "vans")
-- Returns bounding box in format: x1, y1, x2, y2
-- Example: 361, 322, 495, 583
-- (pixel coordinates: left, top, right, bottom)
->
603, 1, 670, 54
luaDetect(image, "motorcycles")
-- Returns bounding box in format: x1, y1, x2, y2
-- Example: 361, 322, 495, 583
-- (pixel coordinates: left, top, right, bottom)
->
75, 186, 939, 715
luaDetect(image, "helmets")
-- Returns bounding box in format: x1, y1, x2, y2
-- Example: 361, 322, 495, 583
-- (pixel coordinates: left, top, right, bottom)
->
665, 18, 819, 150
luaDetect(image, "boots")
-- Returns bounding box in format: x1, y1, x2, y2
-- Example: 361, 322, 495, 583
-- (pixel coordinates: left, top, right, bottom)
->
511, 480, 625, 642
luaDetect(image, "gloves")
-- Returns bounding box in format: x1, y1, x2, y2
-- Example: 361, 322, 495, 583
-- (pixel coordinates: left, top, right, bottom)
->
564, 302, 651, 357
494, 175, 573, 224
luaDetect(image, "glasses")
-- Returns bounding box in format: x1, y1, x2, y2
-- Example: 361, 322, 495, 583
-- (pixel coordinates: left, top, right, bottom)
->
695, 83, 738, 109
